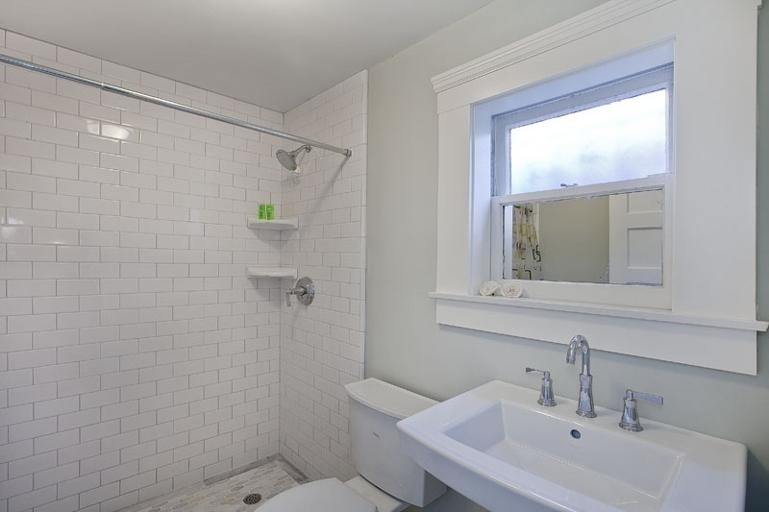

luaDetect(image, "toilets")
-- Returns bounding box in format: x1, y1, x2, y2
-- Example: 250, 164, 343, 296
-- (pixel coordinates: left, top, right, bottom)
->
253, 375, 448, 512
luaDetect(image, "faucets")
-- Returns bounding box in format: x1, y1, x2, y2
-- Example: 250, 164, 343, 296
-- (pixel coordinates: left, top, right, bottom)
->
566, 332, 598, 419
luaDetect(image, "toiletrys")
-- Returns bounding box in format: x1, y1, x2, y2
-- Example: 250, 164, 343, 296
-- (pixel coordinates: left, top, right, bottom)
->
258, 191, 274, 220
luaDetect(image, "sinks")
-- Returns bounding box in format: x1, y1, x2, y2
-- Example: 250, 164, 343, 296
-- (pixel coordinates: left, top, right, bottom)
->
395, 379, 749, 511
